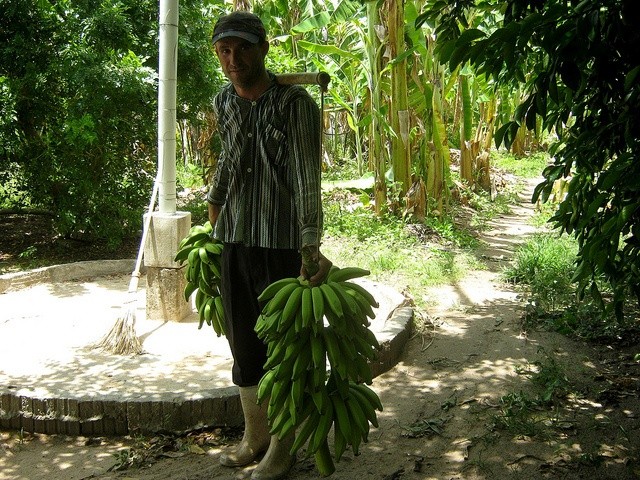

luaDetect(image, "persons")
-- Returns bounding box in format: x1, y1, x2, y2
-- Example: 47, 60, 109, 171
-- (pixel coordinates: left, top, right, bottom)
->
207, 9, 333, 480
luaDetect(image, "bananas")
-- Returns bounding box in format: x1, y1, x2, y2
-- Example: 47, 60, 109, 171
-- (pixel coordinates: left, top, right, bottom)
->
174, 221, 226, 337
253, 267, 383, 464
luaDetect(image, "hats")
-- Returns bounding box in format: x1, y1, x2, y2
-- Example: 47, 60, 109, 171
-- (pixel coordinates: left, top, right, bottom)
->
210, 11, 266, 46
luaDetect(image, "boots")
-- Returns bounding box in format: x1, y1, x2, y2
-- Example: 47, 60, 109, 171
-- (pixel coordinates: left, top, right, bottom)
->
219, 385, 271, 467
251, 426, 296, 479
